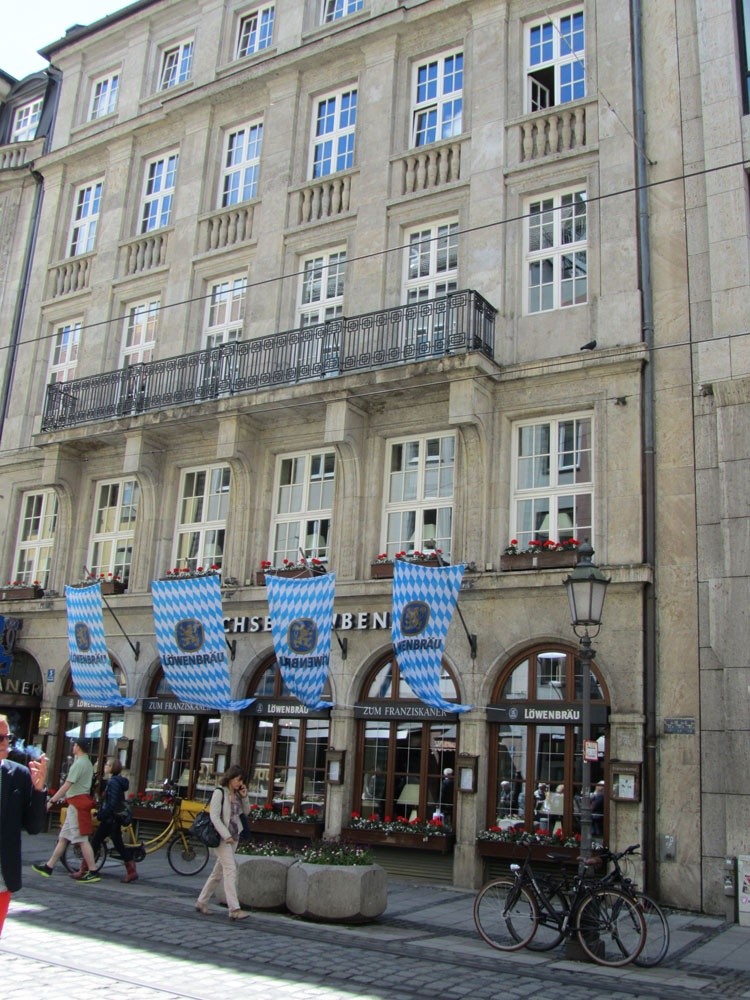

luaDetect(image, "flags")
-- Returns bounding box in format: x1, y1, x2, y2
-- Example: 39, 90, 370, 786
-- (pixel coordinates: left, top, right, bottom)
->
148, 573, 257, 712
262, 570, 337, 712
62, 581, 139, 710
389, 556, 474, 715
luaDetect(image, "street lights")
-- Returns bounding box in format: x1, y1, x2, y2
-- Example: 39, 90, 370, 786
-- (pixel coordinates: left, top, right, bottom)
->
559, 538, 614, 965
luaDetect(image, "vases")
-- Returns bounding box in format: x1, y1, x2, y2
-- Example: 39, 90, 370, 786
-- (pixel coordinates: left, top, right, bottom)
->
255, 570, 315, 585
369, 560, 439, 578
128, 804, 174, 822
246, 816, 325, 837
286, 860, 388, 923
0, 587, 42, 601
478, 839, 598, 861
214, 853, 302, 911
71, 582, 125, 594
498, 551, 580, 571
345, 828, 455, 854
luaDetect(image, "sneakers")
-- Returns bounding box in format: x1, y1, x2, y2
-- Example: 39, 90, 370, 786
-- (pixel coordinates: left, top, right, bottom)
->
76, 871, 101, 883
31, 864, 53, 878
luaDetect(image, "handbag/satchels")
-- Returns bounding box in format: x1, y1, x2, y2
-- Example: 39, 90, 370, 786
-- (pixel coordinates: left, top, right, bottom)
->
188, 788, 224, 847
115, 807, 133, 827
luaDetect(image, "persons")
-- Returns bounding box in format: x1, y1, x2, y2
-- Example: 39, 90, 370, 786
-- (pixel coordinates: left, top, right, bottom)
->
31, 737, 102, 884
67, 755, 141, 884
192, 763, 252, 920
0, 712, 51, 944
361, 765, 605, 843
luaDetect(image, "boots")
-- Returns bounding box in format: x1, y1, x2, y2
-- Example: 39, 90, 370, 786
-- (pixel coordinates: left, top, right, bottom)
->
68, 858, 89, 879
120, 860, 138, 883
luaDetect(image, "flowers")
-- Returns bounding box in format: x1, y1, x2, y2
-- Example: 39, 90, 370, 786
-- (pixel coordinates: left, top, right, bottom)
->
479, 825, 602, 849
504, 539, 579, 552
236, 838, 372, 866
126, 791, 180, 810
166, 565, 220, 576
1, 579, 39, 588
82, 570, 121, 583
249, 802, 318, 824
350, 811, 452, 835
261, 557, 321, 571
377, 549, 442, 560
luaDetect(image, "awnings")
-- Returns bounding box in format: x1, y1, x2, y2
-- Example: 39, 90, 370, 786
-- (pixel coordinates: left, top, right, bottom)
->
65, 720, 160, 744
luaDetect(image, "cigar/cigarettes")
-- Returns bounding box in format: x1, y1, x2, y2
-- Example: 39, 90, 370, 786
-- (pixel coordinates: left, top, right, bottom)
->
38, 755, 49, 762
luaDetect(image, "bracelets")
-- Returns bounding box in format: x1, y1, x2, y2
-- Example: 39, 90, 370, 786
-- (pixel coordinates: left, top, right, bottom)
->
47, 798, 55, 804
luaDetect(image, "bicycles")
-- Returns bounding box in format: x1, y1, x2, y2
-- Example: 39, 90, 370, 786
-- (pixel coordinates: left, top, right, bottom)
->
474, 830, 649, 967
502, 842, 670, 967
57, 778, 211, 878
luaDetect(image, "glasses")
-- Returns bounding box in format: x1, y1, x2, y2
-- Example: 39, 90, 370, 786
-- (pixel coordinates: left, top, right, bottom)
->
0, 733, 14, 743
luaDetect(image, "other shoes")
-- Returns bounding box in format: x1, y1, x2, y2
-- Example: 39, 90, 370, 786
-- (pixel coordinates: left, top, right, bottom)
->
229, 910, 249, 919
195, 902, 214, 915
